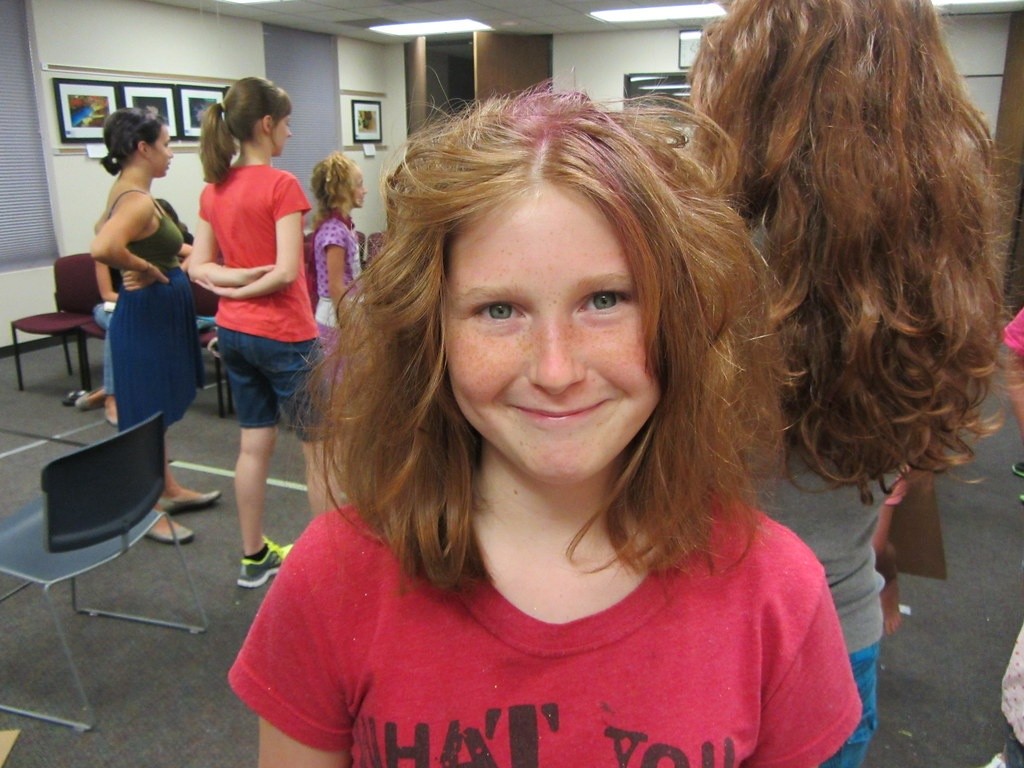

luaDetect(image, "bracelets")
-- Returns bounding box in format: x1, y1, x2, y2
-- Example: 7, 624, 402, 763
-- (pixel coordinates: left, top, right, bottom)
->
141, 260, 150, 274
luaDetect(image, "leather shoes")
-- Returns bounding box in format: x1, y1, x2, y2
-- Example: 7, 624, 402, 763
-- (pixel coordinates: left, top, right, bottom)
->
103, 400, 119, 428
157, 490, 222, 514
75, 386, 106, 411
145, 515, 194, 544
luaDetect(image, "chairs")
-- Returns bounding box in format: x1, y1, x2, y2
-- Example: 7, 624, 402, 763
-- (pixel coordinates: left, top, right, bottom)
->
0, 409, 211, 730
11, 231, 385, 418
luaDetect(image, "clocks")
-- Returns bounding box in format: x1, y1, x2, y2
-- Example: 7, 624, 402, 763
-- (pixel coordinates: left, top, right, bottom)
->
678, 29, 704, 69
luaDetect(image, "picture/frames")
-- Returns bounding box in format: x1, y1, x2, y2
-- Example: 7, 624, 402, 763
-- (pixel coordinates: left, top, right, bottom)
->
52, 77, 122, 144
119, 82, 179, 141
176, 84, 226, 141
351, 99, 382, 143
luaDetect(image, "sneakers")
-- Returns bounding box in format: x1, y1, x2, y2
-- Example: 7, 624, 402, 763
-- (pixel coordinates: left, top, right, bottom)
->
236, 534, 295, 589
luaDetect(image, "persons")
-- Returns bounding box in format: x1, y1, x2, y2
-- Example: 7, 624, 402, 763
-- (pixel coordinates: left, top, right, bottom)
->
309, 151, 368, 380
74, 199, 194, 427
979, 307, 1024, 768
870, 455, 933, 633
187, 76, 340, 589
226, 89, 864, 768
681, 0, 1010, 768
88, 109, 222, 544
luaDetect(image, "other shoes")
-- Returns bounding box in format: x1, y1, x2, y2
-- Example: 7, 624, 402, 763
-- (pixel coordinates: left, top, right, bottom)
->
1012, 462, 1024, 478
